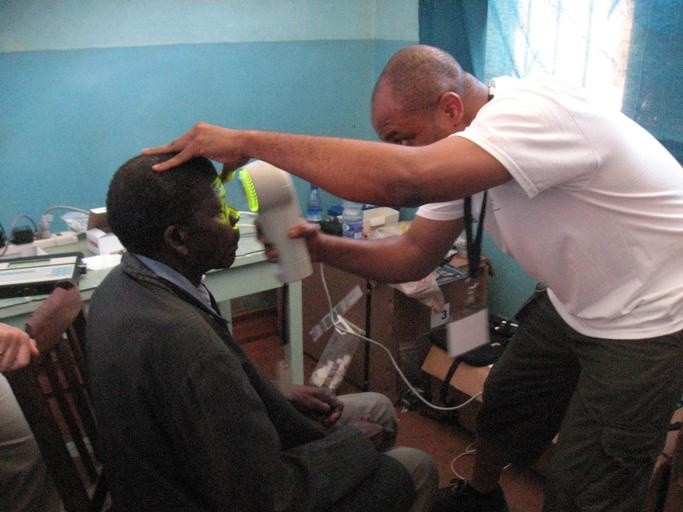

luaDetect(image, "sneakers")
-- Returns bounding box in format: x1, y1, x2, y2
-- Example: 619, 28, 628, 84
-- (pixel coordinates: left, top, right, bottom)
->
440, 480, 510, 511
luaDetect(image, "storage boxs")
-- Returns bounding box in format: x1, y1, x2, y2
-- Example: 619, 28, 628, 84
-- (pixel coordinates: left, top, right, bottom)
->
420, 332, 509, 435
274, 228, 493, 401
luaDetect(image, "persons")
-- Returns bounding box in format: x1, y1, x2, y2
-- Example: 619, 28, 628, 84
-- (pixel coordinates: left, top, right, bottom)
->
82, 149, 439, 511
0, 322, 68, 512
143, 44, 683, 510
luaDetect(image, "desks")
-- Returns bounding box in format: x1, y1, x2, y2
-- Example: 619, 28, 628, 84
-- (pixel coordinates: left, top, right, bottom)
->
0, 214, 304, 457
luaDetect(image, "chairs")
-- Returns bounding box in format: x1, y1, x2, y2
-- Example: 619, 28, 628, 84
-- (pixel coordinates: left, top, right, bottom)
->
3, 277, 111, 511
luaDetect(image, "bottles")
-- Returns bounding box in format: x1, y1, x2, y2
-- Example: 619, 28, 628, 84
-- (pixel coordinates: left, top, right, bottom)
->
306, 183, 322, 223
340, 197, 363, 240
